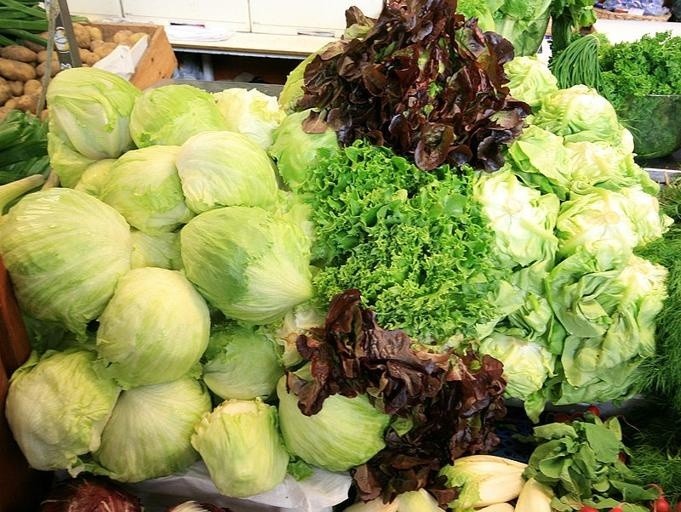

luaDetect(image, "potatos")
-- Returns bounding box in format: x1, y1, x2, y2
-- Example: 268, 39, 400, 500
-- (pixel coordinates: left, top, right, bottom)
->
0, 22, 148, 125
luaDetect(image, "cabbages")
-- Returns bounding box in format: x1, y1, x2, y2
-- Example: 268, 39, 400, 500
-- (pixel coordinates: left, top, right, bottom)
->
0, 41, 671, 511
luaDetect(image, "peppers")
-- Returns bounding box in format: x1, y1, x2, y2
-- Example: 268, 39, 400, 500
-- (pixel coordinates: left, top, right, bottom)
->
0, 1, 88, 52
